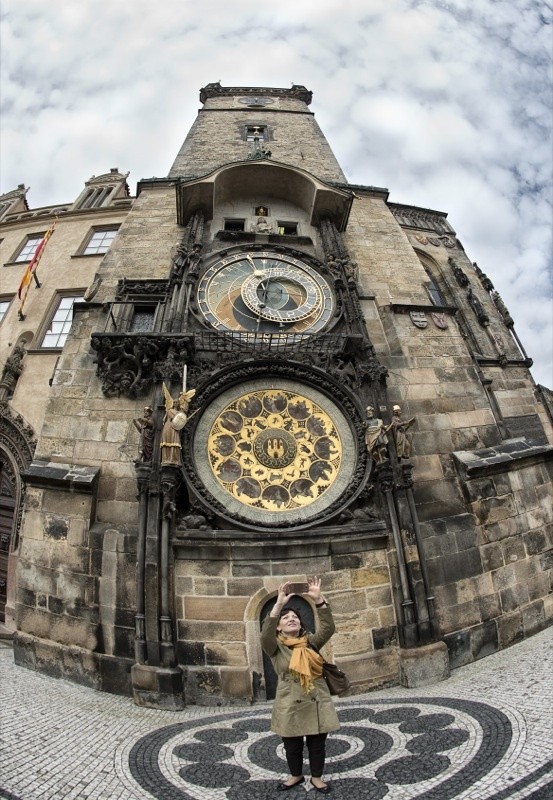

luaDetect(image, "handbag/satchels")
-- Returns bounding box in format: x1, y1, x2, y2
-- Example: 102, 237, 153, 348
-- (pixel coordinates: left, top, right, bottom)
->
308, 642, 352, 695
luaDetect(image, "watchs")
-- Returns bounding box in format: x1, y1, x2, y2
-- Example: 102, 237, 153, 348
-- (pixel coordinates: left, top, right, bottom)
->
314, 599, 328, 609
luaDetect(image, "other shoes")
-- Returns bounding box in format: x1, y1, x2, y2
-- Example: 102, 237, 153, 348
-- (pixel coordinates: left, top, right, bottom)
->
310, 777, 331, 794
278, 777, 305, 791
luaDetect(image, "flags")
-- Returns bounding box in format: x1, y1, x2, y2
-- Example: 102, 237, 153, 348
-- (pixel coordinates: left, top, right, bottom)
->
18, 224, 54, 298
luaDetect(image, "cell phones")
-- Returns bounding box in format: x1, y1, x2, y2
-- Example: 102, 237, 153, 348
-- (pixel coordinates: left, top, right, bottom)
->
285, 583, 310, 594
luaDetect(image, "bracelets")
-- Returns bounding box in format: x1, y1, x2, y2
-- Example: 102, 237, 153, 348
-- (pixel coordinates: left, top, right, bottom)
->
271, 610, 280, 617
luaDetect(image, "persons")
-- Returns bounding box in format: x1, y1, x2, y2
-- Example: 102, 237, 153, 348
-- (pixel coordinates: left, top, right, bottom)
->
362, 405, 390, 465
392, 405, 416, 465
132, 405, 155, 464
251, 217, 273, 235
171, 243, 203, 279
160, 399, 186, 466
260, 576, 350, 795
327, 253, 355, 283
